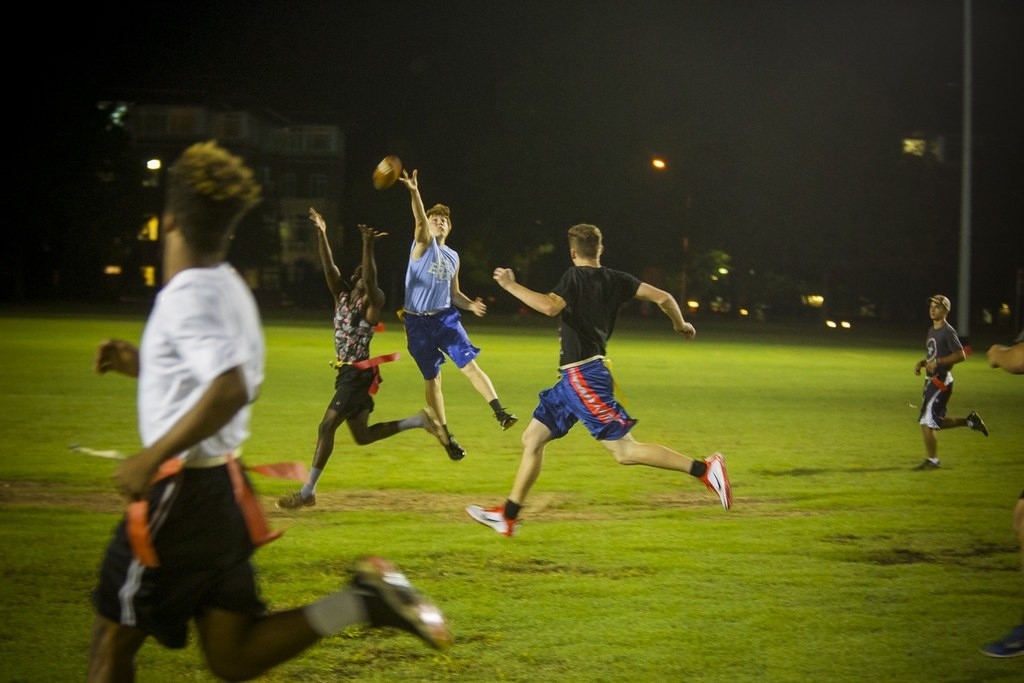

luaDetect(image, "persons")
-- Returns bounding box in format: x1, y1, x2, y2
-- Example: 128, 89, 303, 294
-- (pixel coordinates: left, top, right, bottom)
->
86, 137, 455, 683
393, 168, 519, 458
464, 224, 730, 537
980, 339, 1024, 657
913, 295, 991, 471
275, 206, 449, 511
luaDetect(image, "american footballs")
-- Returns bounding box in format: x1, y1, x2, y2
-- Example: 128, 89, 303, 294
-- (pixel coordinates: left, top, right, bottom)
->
371, 154, 403, 191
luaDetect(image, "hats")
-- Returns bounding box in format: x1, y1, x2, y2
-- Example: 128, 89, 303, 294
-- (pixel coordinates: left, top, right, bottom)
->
928, 294, 950, 311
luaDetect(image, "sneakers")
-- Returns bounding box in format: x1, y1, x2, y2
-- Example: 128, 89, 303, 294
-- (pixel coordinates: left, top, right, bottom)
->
419, 405, 449, 446
466, 504, 523, 537
494, 407, 519, 431
274, 490, 315, 512
977, 624, 1024, 658
349, 555, 448, 649
914, 458, 941, 471
967, 411, 989, 436
699, 452, 733, 511
443, 434, 466, 461
